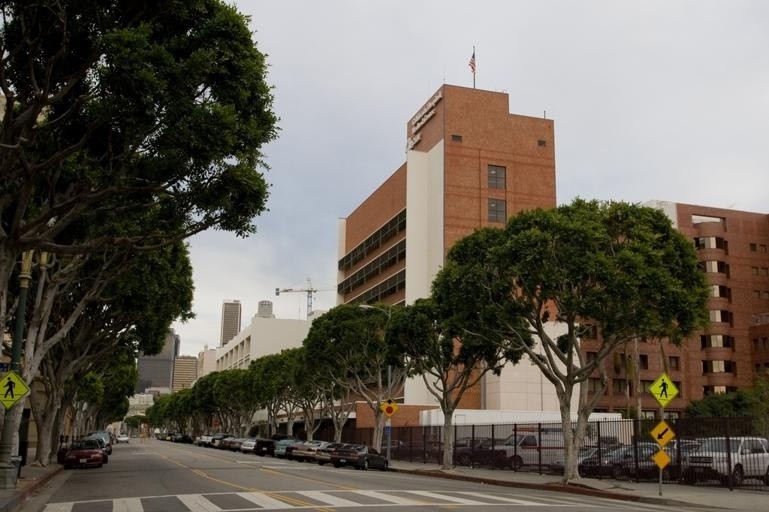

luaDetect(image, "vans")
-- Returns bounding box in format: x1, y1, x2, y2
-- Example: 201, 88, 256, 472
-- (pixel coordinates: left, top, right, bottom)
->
489, 431, 566, 471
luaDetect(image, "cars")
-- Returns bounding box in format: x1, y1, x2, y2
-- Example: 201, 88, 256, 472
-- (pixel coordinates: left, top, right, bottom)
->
154, 433, 388, 472
438, 436, 488, 465
395, 441, 434, 461
669, 438, 705, 461
115, 435, 128, 443
580, 443, 671, 480
469, 438, 505, 469
64, 431, 113, 471
381, 439, 404, 461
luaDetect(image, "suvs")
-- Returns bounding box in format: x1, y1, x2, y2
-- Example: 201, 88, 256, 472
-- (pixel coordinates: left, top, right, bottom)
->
680, 437, 769, 487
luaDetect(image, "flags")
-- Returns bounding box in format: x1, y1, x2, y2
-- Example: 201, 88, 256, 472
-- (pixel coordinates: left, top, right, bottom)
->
468, 53, 477, 74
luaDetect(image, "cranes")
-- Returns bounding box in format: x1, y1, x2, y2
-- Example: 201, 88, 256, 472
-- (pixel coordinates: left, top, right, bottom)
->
275, 278, 316, 314
